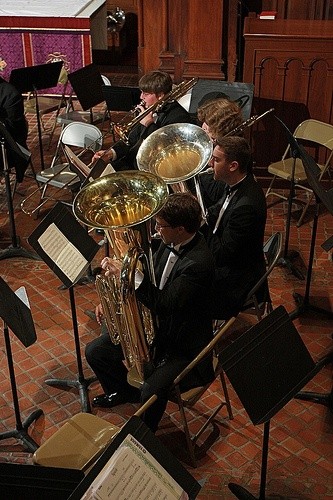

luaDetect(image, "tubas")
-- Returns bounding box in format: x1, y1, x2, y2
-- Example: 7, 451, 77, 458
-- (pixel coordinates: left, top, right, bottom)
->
135, 123, 214, 230
71, 170, 170, 379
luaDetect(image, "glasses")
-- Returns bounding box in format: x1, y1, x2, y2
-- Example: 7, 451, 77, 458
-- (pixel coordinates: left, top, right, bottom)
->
154, 222, 171, 230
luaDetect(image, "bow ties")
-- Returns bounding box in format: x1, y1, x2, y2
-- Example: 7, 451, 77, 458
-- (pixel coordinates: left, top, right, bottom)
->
225, 182, 241, 197
165, 246, 181, 257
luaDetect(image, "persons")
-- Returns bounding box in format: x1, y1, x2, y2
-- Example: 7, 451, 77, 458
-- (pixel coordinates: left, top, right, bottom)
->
0, 78, 32, 183
92, 70, 271, 320
85, 190, 215, 437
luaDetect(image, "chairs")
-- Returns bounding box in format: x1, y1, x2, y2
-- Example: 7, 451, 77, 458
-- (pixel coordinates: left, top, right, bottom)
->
265, 119, 333, 227
35, 122, 103, 218
127, 319, 233, 469
56, 74, 116, 143
24, 66, 69, 151
213, 232, 284, 335
31, 395, 157, 477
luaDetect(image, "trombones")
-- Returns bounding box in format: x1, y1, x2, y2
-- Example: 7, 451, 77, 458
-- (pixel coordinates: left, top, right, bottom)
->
21, 76, 200, 216
220, 107, 276, 138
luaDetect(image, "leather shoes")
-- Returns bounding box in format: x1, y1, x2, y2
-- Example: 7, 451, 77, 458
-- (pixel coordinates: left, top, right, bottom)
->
92, 389, 129, 409
